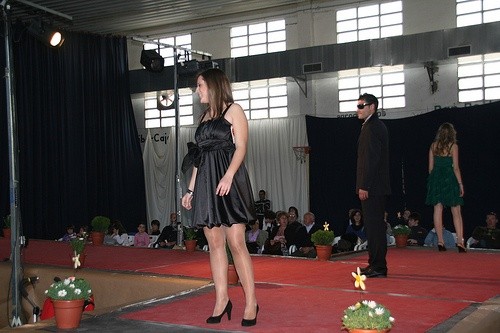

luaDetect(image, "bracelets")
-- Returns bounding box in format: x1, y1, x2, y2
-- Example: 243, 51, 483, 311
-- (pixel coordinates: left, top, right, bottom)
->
459, 182, 464, 185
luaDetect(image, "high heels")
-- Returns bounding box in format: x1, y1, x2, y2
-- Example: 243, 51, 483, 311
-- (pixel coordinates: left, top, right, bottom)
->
206, 299, 232, 323
437, 242, 446, 251
457, 243, 466, 252
241, 304, 258, 326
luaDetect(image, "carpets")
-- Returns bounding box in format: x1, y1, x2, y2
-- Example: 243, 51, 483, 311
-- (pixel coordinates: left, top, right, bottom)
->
0, 238, 500, 333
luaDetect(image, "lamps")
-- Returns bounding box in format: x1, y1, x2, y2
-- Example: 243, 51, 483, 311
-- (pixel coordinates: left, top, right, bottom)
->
139, 42, 165, 73
199, 54, 220, 72
29, 9, 63, 49
182, 50, 199, 72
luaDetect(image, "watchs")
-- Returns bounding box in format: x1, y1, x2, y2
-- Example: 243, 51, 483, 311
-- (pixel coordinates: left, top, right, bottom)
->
187, 188, 195, 196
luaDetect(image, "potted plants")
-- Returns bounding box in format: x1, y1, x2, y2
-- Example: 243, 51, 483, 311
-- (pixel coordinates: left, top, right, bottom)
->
225, 243, 239, 286
90, 215, 110, 246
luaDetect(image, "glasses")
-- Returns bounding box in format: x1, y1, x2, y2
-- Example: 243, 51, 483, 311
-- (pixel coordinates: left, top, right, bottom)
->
357, 104, 371, 109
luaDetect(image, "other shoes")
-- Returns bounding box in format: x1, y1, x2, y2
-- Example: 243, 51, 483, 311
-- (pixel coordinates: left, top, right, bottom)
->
359, 265, 371, 272
361, 270, 387, 278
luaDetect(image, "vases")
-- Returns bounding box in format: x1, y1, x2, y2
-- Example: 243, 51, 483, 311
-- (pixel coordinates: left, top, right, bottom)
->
50, 298, 85, 330
394, 233, 408, 246
315, 244, 334, 262
347, 327, 390, 333
1, 227, 12, 239
184, 239, 197, 251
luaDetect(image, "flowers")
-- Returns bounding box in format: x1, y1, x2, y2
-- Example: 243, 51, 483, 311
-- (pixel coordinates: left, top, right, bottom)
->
183, 224, 198, 240
311, 221, 336, 246
2, 214, 11, 229
340, 266, 396, 330
44, 276, 93, 300
392, 211, 413, 236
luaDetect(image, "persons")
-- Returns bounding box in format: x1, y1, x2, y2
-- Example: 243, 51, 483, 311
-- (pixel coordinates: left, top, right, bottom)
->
470, 212, 500, 248
425, 221, 456, 247
395, 209, 412, 225
77, 225, 93, 243
333, 210, 366, 253
197, 223, 211, 252
151, 220, 161, 236
158, 212, 187, 248
288, 206, 302, 231
289, 211, 320, 258
244, 218, 268, 254
259, 210, 278, 238
134, 222, 150, 247
262, 212, 297, 255
61, 224, 77, 242
110, 220, 128, 246
354, 93, 390, 278
254, 190, 271, 230
383, 211, 391, 245
407, 214, 428, 244
424, 123, 468, 252
181, 68, 259, 327
